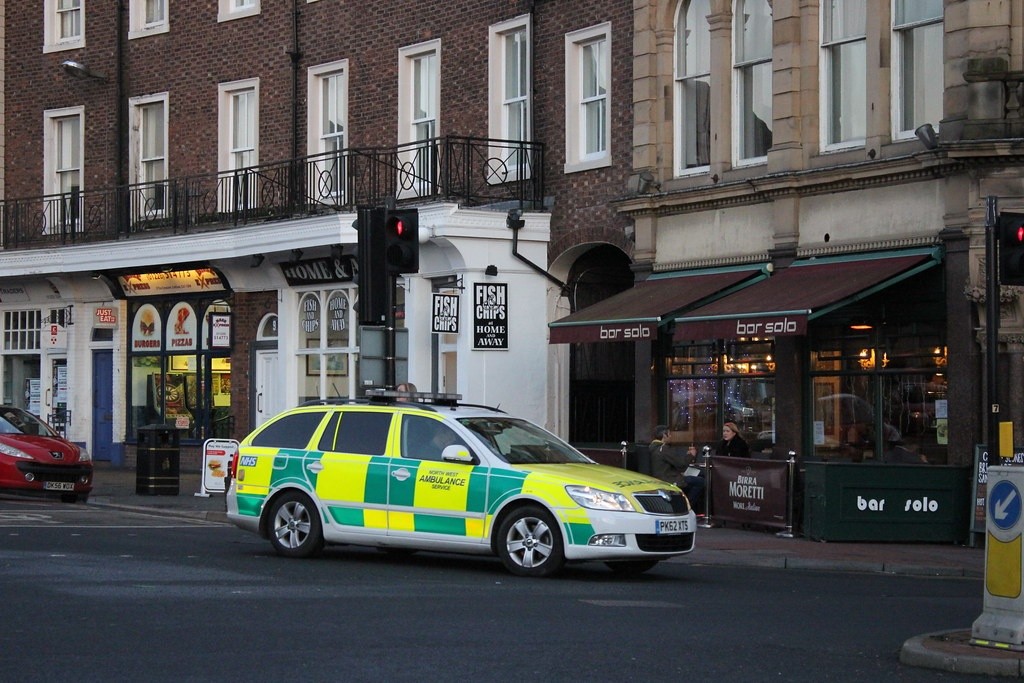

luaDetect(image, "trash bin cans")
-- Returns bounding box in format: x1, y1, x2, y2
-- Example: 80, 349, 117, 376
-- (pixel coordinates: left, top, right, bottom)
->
134, 424, 182, 496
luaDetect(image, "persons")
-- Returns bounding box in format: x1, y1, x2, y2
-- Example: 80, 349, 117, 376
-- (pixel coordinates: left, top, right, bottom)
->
396, 382, 419, 402
648, 424, 705, 515
885, 436, 929, 465
415, 422, 456, 461
716, 421, 752, 458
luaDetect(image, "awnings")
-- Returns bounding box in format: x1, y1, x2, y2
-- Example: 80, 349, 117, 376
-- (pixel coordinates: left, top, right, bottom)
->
672, 245, 942, 342
547, 262, 774, 345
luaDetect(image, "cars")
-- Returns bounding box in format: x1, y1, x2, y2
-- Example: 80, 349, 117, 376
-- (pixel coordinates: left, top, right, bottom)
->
0, 400, 98, 506
224, 379, 703, 577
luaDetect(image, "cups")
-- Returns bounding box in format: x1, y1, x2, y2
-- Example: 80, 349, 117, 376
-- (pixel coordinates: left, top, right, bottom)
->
689, 447, 695, 451
